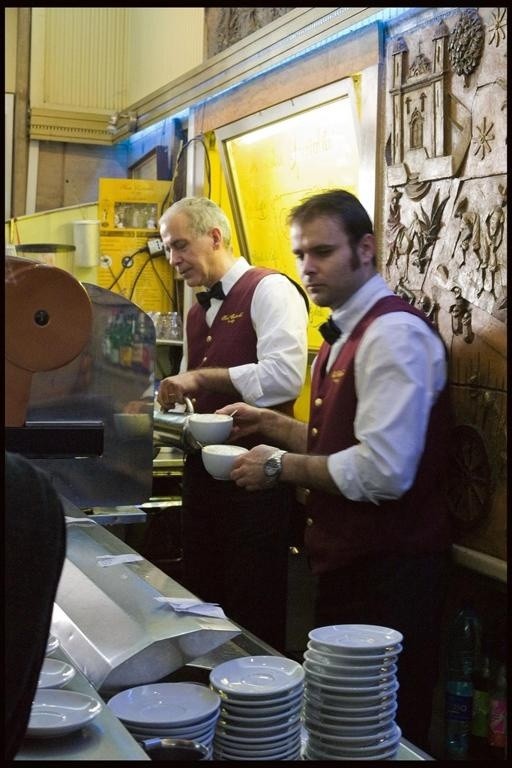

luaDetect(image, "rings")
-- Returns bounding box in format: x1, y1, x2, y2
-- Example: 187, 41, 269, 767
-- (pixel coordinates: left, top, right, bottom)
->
168, 392, 177, 398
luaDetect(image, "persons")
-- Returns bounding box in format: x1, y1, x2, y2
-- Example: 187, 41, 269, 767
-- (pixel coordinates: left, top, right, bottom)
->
151, 196, 311, 652
214, 189, 451, 747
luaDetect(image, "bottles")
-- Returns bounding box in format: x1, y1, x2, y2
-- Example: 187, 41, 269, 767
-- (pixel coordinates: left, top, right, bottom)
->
145, 311, 183, 342
440, 599, 507, 759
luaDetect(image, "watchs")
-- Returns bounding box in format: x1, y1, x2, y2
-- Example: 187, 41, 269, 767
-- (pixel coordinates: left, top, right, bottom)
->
264, 449, 288, 490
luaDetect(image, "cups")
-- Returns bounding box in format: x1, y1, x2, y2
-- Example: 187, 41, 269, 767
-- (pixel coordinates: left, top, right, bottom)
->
179, 409, 237, 448
198, 438, 251, 484
73, 219, 100, 268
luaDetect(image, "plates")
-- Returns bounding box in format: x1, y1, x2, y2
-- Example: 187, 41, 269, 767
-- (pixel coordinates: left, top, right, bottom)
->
207, 655, 307, 764
21, 631, 102, 741
106, 681, 221, 751
301, 622, 403, 762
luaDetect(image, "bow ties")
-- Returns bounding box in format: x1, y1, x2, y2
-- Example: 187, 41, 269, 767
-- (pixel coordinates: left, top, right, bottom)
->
318, 317, 343, 345
194, 281, 226, 311
